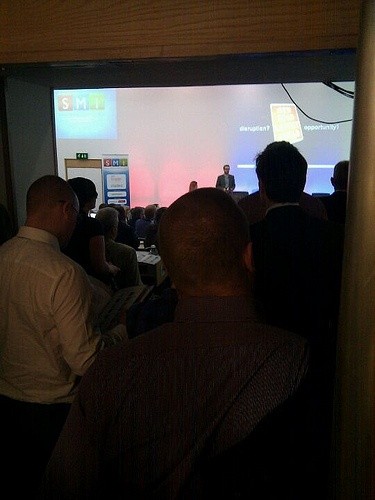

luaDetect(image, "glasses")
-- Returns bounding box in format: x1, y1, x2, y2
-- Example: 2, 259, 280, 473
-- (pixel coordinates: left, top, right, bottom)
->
56, 200, 83, 224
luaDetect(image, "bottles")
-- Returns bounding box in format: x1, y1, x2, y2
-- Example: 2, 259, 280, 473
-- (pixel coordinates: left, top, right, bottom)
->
150, 245, 157, 255
138, 241, 145, 252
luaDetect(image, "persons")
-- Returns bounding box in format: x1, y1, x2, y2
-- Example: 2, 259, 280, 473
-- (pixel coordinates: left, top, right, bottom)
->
39, 187, 335, 500
0, 141, 349, 500
